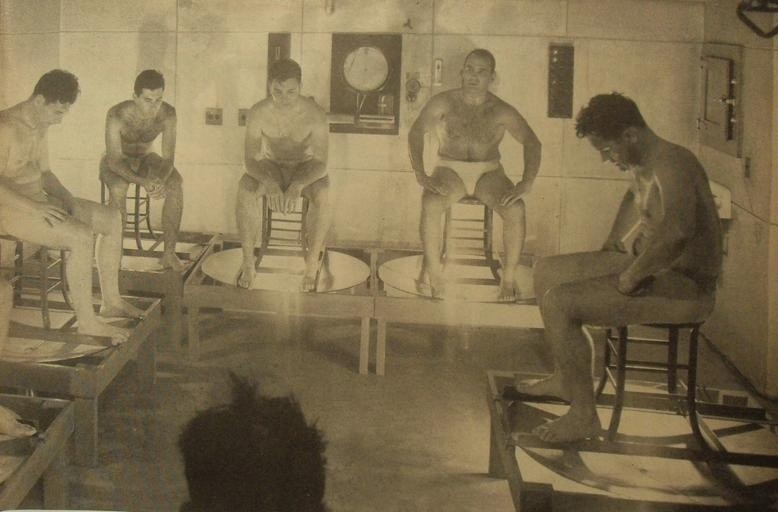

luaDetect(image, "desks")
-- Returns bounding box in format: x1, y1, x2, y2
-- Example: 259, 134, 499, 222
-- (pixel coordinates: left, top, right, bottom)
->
482, 364, 777, 512
1, 233, 213, 512
180, 236, 545, 374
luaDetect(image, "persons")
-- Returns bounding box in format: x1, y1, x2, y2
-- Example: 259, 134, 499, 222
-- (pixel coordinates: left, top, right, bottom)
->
407, 49, 543, 302
515, 89, 723, 443
99, 67, 187, 273
174, 368, 329, 511
233, 58, 336, 292
0, 67, 150, 349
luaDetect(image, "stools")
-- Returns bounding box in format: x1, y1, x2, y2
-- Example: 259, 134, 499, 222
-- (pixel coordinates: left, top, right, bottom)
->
256, 194, 309, 268
596, 322, 718, 455
99, 173, 158, 256
440, 196, 501, 284
